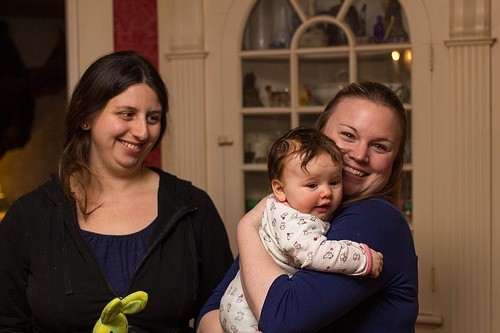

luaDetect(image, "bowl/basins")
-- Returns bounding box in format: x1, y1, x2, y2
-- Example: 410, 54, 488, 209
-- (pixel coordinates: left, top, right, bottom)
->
308, 81, 346, 105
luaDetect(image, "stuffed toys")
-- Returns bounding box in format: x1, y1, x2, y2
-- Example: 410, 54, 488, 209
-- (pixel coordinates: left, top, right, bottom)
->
93, 291, 148, 333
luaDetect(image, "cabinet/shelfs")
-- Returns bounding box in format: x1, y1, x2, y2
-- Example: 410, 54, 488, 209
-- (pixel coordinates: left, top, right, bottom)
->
242, 0, 412, 227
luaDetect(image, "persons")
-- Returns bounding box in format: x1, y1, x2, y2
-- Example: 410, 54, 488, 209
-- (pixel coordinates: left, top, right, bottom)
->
194, 81, 419, 333
218, 125, 383, 333
0, 50, 235, 333
